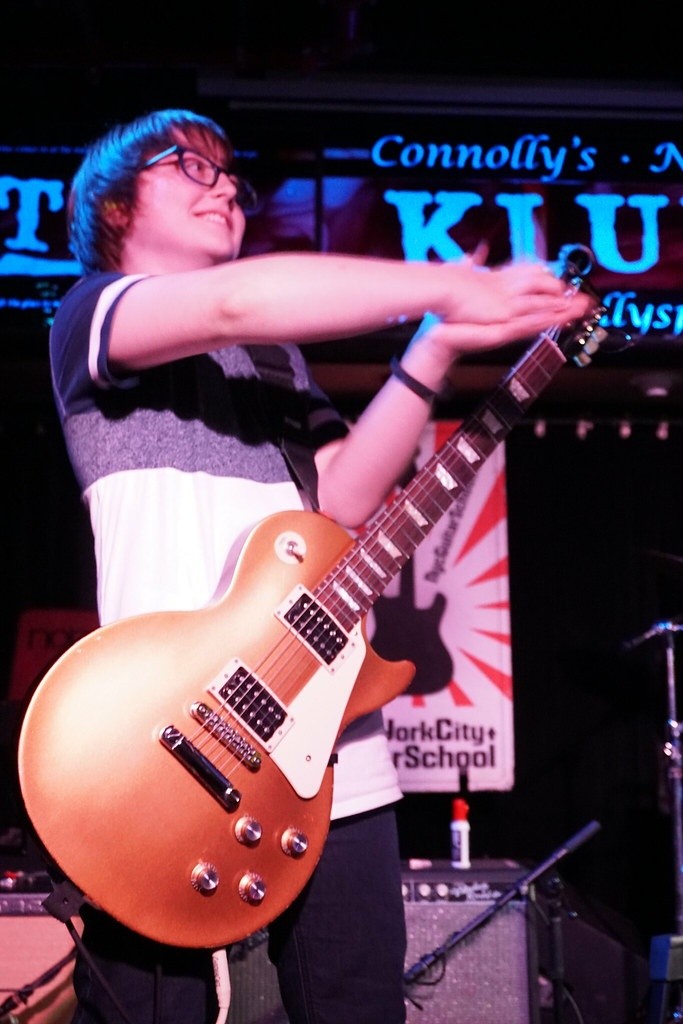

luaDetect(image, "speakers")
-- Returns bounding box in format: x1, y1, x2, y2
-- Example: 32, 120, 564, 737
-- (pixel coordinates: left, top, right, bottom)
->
1, 857, 653, 1023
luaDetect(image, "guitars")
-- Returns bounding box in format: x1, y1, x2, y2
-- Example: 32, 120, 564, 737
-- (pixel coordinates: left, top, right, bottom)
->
15, 273, 614, 955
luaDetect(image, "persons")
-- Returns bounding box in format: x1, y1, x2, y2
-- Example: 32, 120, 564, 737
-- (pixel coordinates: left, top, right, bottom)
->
48, 109, 590, 1024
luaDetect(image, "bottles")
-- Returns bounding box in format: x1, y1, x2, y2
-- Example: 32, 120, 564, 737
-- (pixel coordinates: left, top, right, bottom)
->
450, 799, 471, 869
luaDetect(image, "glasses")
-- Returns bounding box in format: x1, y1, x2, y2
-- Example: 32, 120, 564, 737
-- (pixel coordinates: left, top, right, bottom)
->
135, 144, 257, 210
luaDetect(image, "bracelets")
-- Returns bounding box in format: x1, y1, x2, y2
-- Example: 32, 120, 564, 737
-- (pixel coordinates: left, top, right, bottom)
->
389, 356, 439, 402
